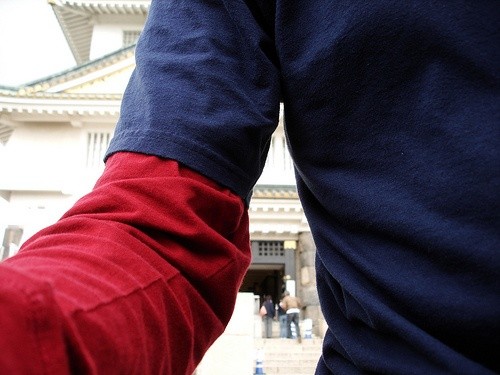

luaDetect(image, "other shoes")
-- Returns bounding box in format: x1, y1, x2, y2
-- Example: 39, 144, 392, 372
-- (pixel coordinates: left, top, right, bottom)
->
297, 336, 302, 343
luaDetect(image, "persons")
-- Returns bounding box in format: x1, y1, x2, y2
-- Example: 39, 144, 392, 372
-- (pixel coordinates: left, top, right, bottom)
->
259, 275, 302, 343
0, 0, 500, 375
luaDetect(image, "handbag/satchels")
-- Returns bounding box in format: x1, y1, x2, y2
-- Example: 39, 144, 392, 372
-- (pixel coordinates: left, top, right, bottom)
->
259, 305, 267, 316
